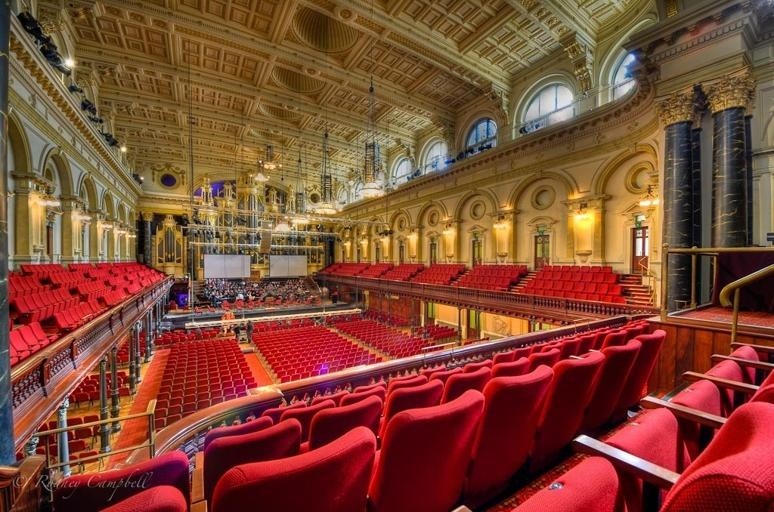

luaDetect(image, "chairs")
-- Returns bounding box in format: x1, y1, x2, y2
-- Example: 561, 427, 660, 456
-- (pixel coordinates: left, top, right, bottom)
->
2, 260, 774, 512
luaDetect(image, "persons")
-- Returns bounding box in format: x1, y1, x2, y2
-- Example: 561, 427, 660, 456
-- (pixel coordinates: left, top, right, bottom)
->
231, 323, 241, 341
246, 321, 252, 344
203, 277, 338, 305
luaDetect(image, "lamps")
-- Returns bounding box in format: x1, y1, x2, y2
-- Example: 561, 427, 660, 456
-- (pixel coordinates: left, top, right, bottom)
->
33, 181, 62, 208
491, 215, 511, 230
197, 2, 394, 238
636, 183, 661, 207
15, 10, 120, 152
441, 222, 453, 235
574, 202, 595, 222
690, 85, 712, 112
406, 117, 541, 188
77, 201, 139, 239
